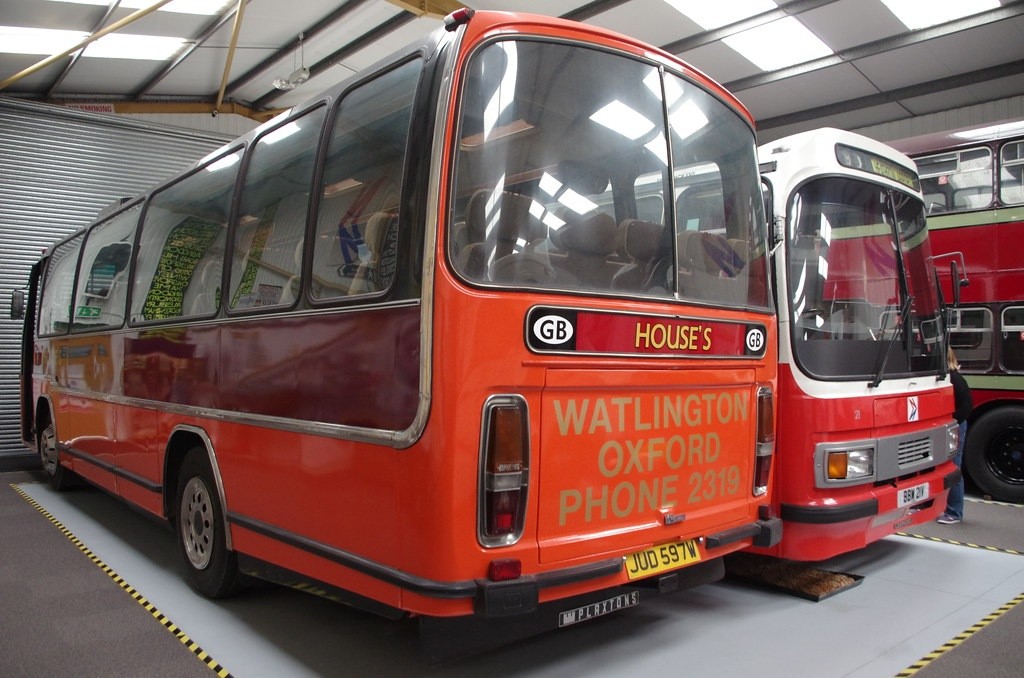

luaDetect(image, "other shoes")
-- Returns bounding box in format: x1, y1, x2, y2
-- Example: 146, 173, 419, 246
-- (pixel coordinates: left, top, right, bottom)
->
938, 512, 961, 525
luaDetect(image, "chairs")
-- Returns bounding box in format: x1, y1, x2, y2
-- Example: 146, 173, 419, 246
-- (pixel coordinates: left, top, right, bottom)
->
192, 188, 751, 309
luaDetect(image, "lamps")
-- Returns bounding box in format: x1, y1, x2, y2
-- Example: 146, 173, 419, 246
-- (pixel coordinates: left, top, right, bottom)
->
273, 66, 311, 90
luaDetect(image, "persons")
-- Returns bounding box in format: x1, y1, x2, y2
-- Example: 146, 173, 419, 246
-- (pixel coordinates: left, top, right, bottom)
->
937, 343, 973, 524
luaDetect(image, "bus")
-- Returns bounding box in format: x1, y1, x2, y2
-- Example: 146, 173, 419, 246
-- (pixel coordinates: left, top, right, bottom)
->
11, 6, 1024, 627
